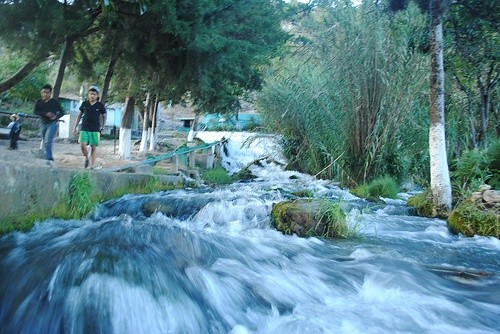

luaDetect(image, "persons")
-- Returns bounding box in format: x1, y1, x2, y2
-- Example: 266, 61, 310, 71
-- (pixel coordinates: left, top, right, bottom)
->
73, 85, 107, 170
7, 114, 22, 150
33, 84, 65, 165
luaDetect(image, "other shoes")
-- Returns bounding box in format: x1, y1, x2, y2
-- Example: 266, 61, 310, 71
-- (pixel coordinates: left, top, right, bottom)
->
84, 160, 89, 168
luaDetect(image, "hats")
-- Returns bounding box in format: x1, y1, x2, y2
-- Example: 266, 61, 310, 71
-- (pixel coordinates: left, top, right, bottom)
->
10, 114, 20, 121
89, 86, 99, 94
